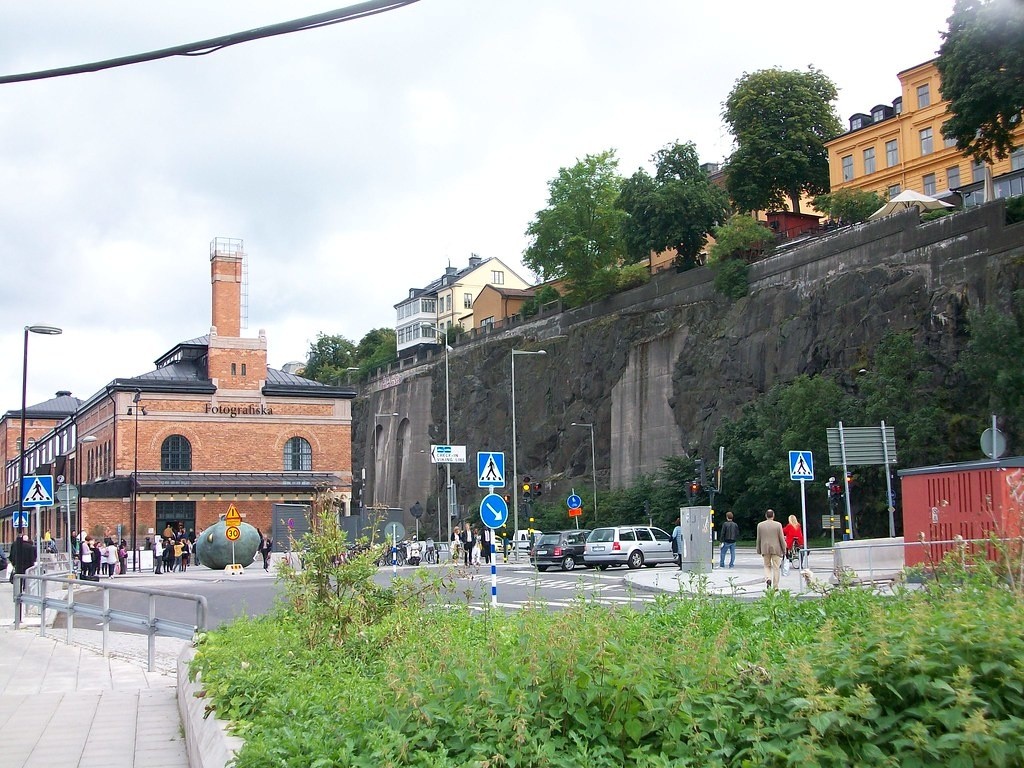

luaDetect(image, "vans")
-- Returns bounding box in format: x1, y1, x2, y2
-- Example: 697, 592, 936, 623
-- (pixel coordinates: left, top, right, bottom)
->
583, 525, 681, 571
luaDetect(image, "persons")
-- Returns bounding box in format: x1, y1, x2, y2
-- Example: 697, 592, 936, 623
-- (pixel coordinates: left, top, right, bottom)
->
44, 529, 51, 542
71, 531, 76, 574
151, 524, 204, 574
823, 218, 836, 229
81, 537, 128, 579
45, 546, 58, 553
104, 526, 111, 545
720, 512, 738, 569
427, 536, 435, 561
756, 509, 786, 591
258, 534, 272, 569
0, 544, 7, 571
671, 518, 683, 570
10, 536, 37, 615
782, 515, 804, 562
449, 523, 491, 566
76, 528, 87, 542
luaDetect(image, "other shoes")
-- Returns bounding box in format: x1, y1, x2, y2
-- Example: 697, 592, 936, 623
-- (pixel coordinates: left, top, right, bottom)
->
109, 575, 114, 580
155, 571, 163, 574
453, 563, 460, 567
465, 563, 468, 566
716, 566, 725, 569
766, 580, 771, 589
469, 563, 473, 565
729, 566, 734, 569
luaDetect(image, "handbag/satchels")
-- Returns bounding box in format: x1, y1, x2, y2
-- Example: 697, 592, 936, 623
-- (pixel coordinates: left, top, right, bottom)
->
253, 551, 260, 562
478, 543, 483, 550
5, 563, 16, 584
481, 548, 486, 557
781, 554, 789, 576
115, 563, 121, 573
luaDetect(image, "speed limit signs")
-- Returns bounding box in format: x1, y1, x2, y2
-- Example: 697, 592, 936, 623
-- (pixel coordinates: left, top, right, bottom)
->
226, 526, 240, 541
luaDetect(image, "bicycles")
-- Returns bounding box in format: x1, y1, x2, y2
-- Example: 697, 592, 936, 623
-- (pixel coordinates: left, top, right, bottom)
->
771, 536, 803, 569
377, 549, 439, 566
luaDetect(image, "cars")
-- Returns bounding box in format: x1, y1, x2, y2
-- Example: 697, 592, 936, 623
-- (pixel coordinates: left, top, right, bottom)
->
479, 530, 544, 550
530, 529, 622, 571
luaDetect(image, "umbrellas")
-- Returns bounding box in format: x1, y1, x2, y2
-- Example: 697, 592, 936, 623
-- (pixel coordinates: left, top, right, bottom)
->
868, 189, 955, 219
983, 166, 996, 203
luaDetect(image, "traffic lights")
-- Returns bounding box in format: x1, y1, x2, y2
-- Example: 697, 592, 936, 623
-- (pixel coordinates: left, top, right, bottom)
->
522, 475, 530, 499
503, 494, 510, 509
691, 458, 718, 495
531, 483, 541, 499
830, 485, 841, 502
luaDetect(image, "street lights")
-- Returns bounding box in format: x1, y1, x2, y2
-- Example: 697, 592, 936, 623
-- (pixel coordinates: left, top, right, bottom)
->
421, 322, 453, 564
512, 348, 546, 562
13, 326, 62, 623
375, 412, 399, 505
78, 406, 147, 571
571, 423, 597, 521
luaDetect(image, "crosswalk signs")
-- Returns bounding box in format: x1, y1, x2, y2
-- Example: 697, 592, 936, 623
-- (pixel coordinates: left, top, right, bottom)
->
22, 476, 54, 507
477, 452, 505, 488
788, 451, 814, 480
13, 511, 28, 528
